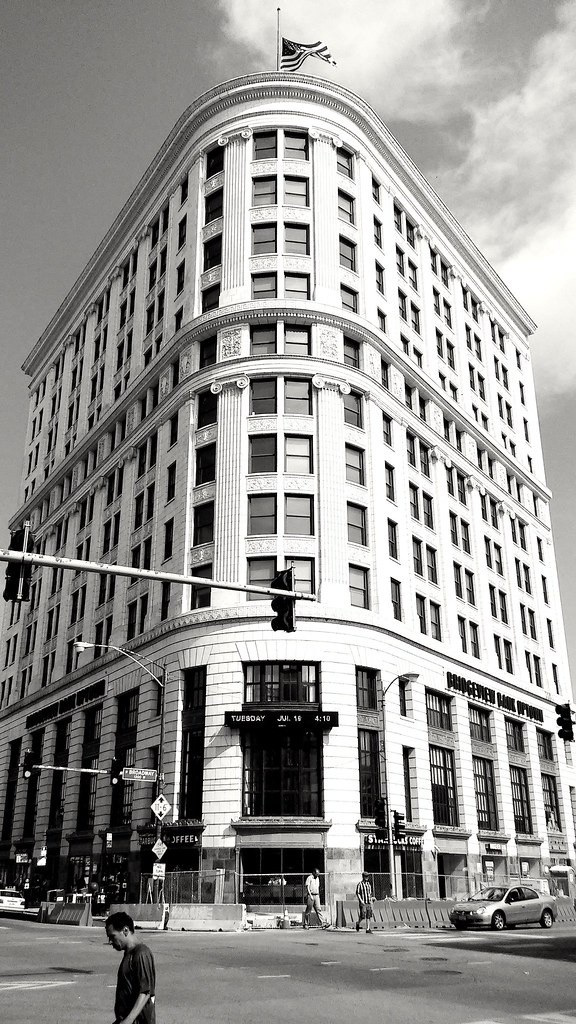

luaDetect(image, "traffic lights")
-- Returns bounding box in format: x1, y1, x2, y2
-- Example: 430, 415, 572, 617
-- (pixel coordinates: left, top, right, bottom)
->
555, 703, 573, 741
22, 750, 35, 779
270, 571, 296, 634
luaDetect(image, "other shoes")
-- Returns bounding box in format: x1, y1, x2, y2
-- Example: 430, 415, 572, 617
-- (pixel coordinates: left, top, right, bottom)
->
322, 924, 330, 930
356, 922, 359, 932
303, 925, 309, 930
366, 929, 372, 933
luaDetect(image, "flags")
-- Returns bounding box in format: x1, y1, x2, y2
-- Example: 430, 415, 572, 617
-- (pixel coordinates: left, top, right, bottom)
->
281, 36, 335, 78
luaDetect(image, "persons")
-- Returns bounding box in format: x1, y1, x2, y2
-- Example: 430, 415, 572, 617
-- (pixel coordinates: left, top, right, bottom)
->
303, 868, 330, 930
356, 872, 376, 933
105, 911, 156, 1024
266, 875, 287, 904
12, 871, 57, 909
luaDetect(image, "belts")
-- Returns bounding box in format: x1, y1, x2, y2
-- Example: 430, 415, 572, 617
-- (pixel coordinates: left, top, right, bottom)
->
308, 893, 318, 895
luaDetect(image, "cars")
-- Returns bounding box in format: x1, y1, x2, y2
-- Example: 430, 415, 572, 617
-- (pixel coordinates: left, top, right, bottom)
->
0, 889, 25, 917
448, 885, 559, 930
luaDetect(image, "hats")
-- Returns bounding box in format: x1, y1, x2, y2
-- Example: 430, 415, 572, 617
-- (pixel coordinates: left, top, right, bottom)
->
362, 872, 369, 877
